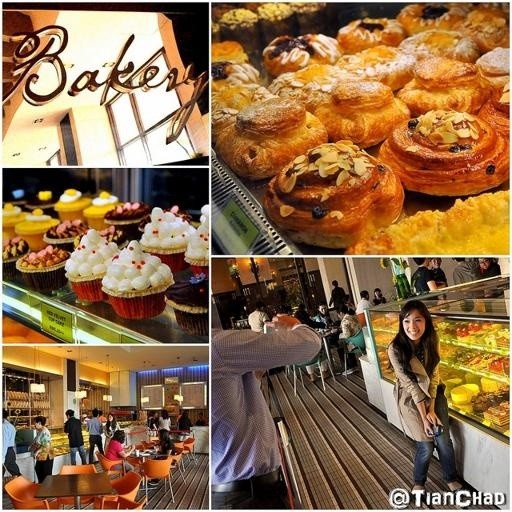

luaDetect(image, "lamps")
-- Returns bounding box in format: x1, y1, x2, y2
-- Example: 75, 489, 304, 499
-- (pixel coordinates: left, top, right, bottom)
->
29, 346, 45, 394
173, 355, 184, 402
73, 348, 87, 399
248, 257, 261, 283
140, 362, 149, 403
102, 355, 113, 402
229, 262, 243, 287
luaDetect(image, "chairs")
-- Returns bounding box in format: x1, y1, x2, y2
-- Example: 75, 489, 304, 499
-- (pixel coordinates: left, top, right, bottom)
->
284, 307, 367, 398
3, 437, 196, 510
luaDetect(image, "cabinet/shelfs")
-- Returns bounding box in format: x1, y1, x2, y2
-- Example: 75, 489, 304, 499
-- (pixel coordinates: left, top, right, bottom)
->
34, 423, 150, 482
364, 272, 510, 509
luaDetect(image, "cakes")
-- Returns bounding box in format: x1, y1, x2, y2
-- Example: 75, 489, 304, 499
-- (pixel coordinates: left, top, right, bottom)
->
210, 3, 510, 253
372, 314, 510, 436
3, 188, 209, 336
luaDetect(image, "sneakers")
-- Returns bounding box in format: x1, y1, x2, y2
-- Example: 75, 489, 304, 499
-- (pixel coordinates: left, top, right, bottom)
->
148, 480, 160, 489
310, 367, 360, 381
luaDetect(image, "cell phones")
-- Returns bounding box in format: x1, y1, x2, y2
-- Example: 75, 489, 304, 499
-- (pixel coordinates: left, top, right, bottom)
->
428, 424, 441, 437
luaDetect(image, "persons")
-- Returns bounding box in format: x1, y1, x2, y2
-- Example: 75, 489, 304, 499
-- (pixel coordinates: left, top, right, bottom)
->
247, 279, 386, 382
63, 409, 87, 465
104, 412, 121, 454
146, 427, 175, 486
86, 407, 104, 464
147, 410, 208, 442
385, 298, 467, 501
410, 258, 502, 293
2, 408, 18, 475
28, 416, 54, 483
98, 410, 107, 422
211, 312, 322, 508
105, 429, 136, 472
81, 413, 90, 423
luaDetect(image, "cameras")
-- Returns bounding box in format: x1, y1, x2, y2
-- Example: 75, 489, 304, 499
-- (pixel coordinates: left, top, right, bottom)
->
264, 321, 276, 334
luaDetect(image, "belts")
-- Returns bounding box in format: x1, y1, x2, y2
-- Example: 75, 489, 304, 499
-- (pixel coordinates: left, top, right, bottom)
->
212, 468, 283, 493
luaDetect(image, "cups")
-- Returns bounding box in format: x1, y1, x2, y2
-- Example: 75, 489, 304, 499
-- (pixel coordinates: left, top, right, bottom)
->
135, 450, 139, 458
137, 242, 188, 275
3, 187, 153, 262
183, 252, 209, 280
100, 275, 174, 323
63, 272, 107, 302
163, 296, 209, 338
3, 244, 29, 281
13, 252, 69, 294
